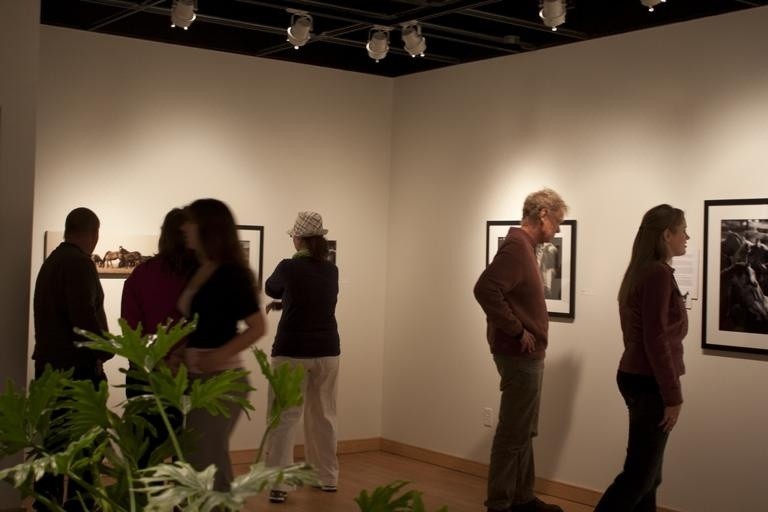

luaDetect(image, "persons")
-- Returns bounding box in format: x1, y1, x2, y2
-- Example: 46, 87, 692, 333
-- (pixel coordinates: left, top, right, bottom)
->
120, 205, 196, 480
262, 210, 341, 502
29, 206, 111, 512
593, 201, 692, 512
471, 186, 565, 510
165, 197, 269, 512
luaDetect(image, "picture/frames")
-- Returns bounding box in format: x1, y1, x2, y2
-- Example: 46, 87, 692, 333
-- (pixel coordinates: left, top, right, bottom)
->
234, 222, 265, 292
483, 218, 578, 320
699, 195, 768, 357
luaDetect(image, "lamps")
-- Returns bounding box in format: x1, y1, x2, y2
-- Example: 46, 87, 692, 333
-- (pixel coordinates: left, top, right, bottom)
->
164, 0, 566, 64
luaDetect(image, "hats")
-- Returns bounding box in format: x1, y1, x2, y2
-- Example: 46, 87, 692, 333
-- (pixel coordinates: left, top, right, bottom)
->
286, 211, 328, 238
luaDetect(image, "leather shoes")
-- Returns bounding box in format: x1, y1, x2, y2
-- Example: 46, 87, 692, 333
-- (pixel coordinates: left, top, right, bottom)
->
511, 497, 563, 511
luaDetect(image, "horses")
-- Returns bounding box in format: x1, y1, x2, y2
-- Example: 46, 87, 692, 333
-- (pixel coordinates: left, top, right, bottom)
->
103, 251, 119, 267
120, 251, 141, 268
92, 254, 102, 265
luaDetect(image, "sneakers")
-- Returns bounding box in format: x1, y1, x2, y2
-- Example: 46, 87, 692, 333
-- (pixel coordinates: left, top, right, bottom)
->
311, 480, 337, 491
269, 490, 288, 502
